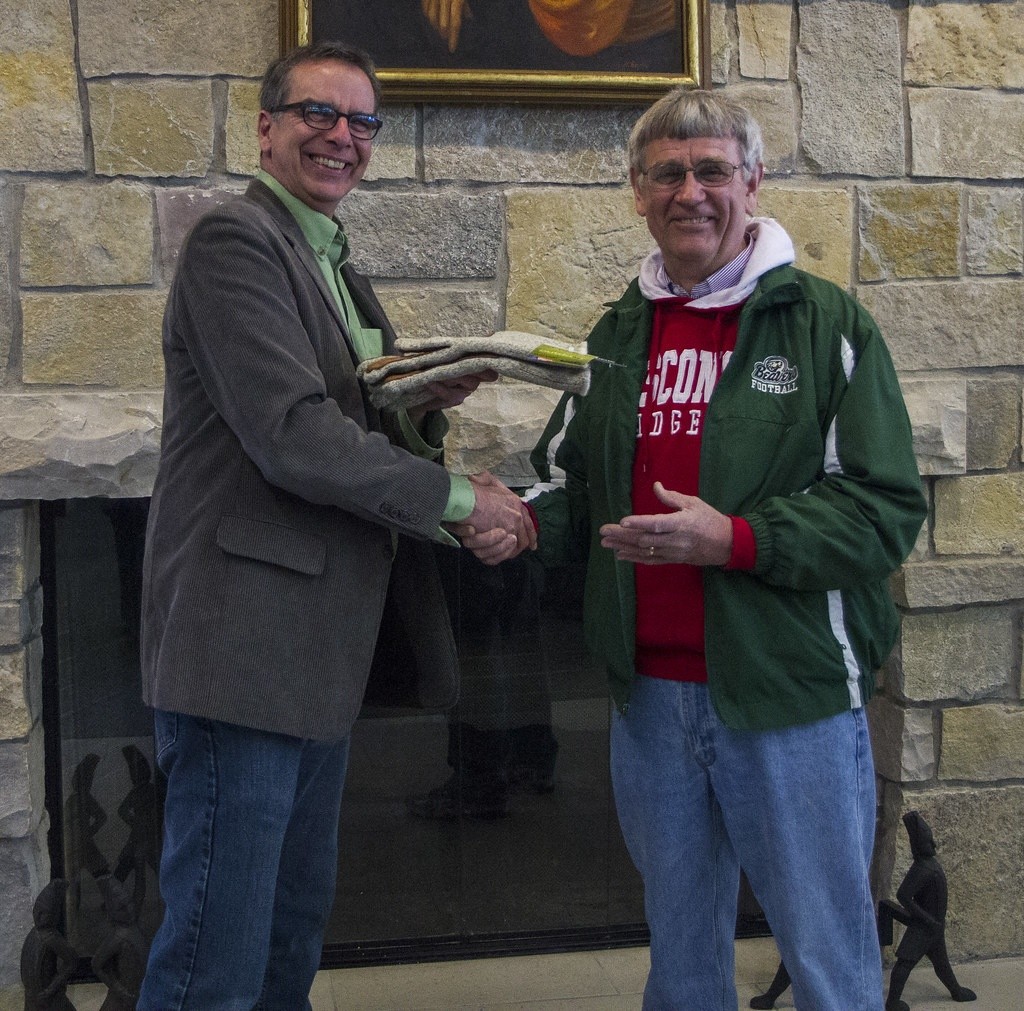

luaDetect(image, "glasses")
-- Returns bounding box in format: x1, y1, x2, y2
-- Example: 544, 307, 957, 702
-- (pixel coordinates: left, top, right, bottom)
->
268, 100, 384, 142
639, 154, 746, 189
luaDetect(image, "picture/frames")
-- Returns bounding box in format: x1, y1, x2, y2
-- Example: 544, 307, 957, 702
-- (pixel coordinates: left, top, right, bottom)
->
278, 0, 712, 106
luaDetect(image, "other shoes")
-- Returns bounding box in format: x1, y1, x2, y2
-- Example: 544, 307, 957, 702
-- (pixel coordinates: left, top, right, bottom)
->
405, 780, 510, 821
478, 749, 559, 792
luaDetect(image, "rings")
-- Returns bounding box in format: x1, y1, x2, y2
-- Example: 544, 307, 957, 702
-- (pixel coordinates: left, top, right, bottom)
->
647, 547, 655, 556
468, 387, 477, 392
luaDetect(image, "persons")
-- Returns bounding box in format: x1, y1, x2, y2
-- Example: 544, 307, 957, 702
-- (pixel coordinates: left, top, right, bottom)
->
132, 38, 537, 1011
457, 86, 932, 1011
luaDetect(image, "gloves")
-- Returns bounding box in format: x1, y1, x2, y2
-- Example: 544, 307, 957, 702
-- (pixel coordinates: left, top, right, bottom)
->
355, 331, 593, 411
